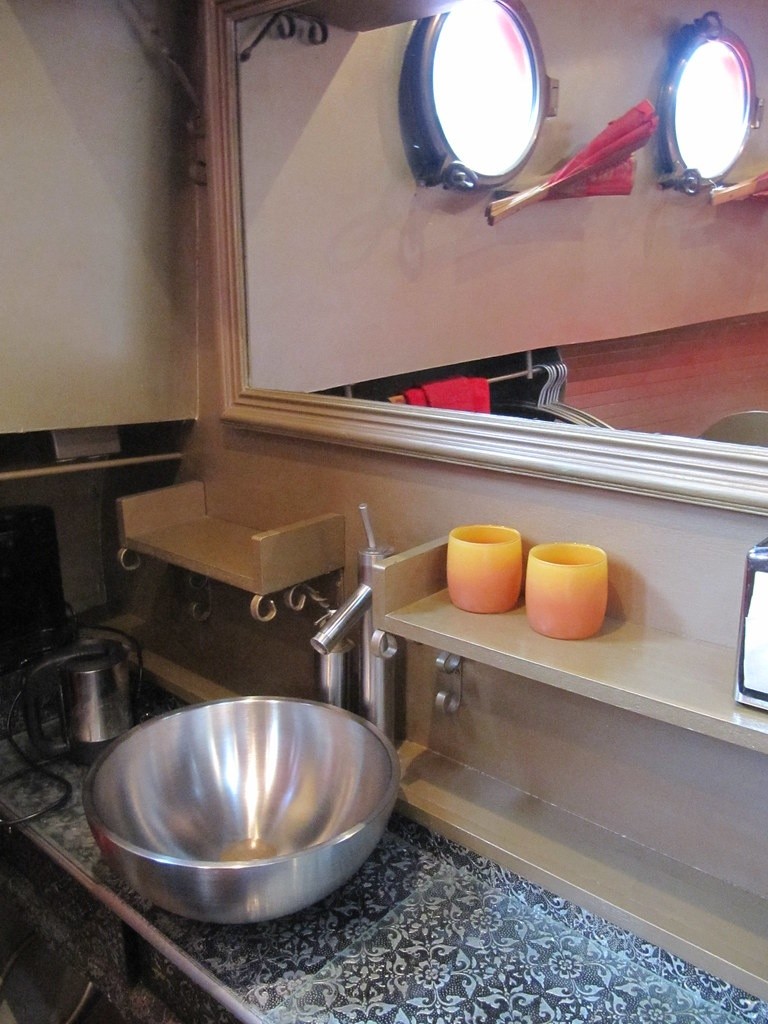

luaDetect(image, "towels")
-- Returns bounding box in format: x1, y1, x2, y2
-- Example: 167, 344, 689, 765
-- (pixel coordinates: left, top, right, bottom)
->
405, 377, 490, 415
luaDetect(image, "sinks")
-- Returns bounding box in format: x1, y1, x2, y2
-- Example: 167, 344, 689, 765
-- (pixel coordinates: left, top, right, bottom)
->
82, 696, 402, 925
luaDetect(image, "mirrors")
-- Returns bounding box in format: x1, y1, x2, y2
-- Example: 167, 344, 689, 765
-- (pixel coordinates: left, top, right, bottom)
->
656, 9, 764, 194
400, 0, 559, 188
200, 0, 768, 518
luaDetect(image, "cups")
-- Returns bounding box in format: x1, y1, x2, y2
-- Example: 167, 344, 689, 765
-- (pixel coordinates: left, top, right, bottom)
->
444, 525, 524, 614
524, 542, 609, 639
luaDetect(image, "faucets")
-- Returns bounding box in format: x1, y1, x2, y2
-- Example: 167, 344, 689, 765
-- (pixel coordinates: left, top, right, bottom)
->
310, 503, 395, 746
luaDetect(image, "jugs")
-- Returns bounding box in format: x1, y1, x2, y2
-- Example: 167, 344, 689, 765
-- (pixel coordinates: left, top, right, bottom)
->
20, 637, 141, 767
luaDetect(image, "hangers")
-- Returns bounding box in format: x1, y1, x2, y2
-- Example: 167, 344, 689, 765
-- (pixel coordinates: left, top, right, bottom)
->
492, 362, 616, 431
